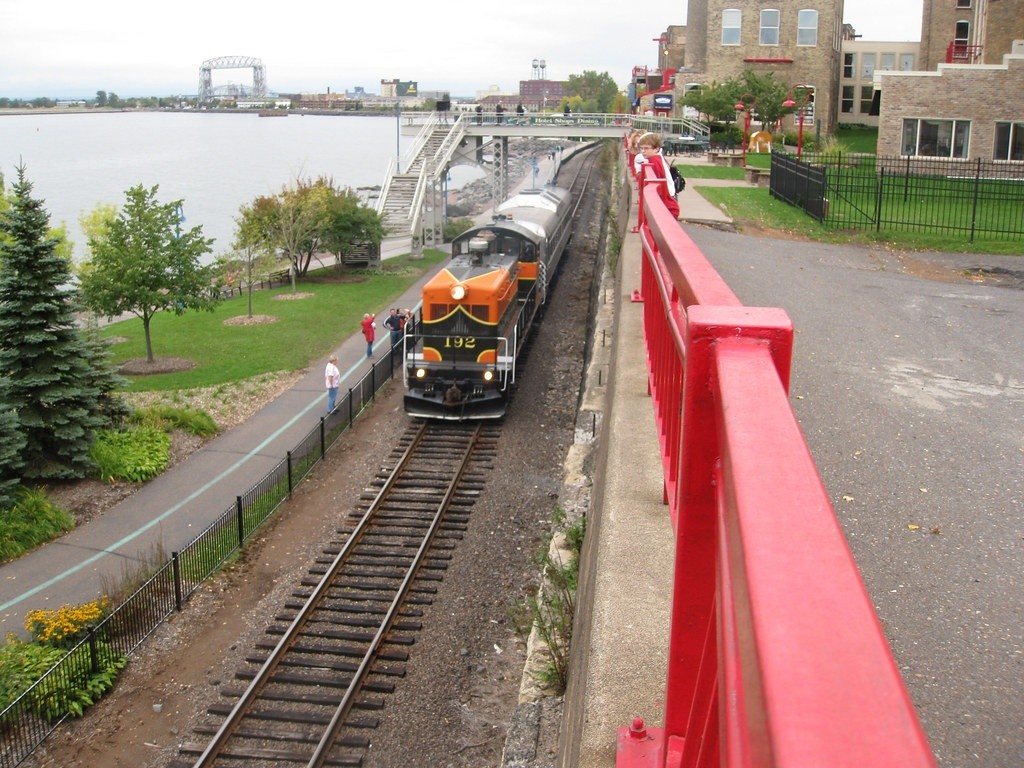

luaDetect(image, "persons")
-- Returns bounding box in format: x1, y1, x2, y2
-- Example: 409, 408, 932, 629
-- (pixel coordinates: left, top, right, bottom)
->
921, 144, 931, 153
903, 143, 915, 156
325, 354, 341, 415
495, 100, 503, 126
361, 313, 375, 359
937, 139, 949, 156
515, 100, 525, 126
621, 123, 687, 234
562, 101, 573, 126
474, 104, 483, 127
381, 307, 412, 355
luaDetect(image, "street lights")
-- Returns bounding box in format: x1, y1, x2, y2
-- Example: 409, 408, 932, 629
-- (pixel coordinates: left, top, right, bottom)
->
781, 82, 811, 160
734, 93, 756, 167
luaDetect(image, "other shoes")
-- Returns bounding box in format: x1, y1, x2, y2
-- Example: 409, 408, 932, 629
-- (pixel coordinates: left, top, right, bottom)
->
368, 355, 374, 358
328, 411, 337, 415
335, 410, 340, 412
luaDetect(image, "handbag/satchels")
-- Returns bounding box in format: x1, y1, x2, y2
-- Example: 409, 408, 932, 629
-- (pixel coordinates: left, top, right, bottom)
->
663, 157, 685, 192
372, 322, 376, 328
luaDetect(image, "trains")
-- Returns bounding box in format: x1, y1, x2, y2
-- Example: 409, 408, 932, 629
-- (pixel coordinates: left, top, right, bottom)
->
397, 183, 574, 425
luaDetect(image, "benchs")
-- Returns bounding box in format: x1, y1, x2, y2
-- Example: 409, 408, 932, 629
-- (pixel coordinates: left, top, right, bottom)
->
708, 153, 770, 185
261, 268, 290, 289
213, 279, 242, 299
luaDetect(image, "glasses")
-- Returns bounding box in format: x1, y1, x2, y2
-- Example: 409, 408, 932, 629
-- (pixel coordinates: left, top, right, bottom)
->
639, 146, 655, 151
